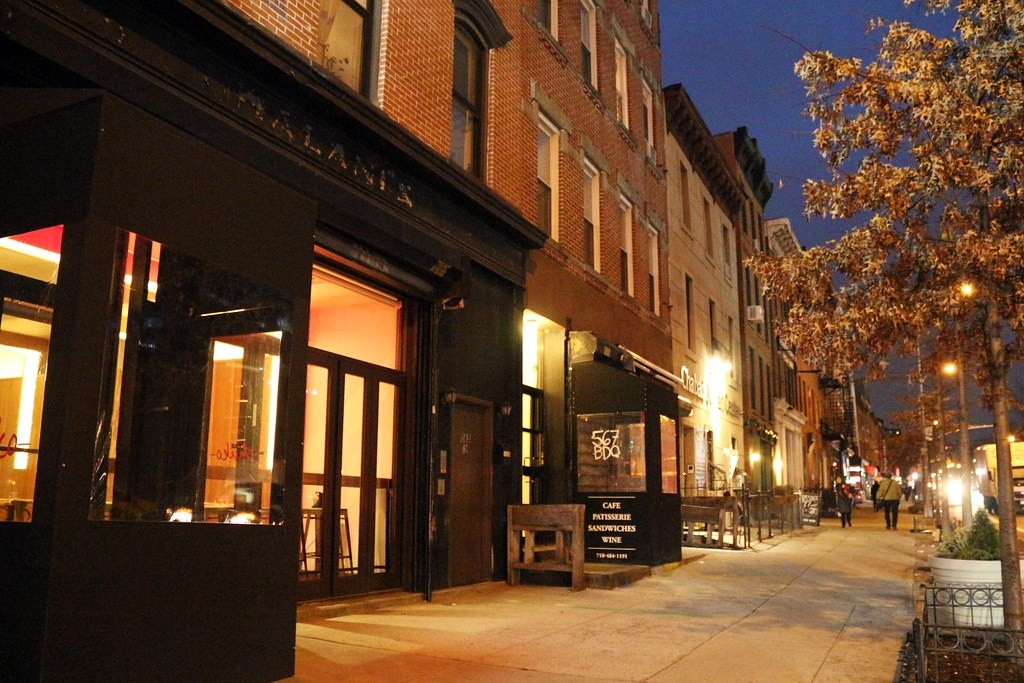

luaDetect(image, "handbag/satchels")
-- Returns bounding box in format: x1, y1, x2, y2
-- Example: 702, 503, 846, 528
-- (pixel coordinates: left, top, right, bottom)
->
851, 498, 856, 507
876, 499, 884, 511
838, 512, 842, 518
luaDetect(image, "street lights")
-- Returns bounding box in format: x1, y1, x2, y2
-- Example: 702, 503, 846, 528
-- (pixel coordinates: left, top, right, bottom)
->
957, 281, 1024, 652
941, 360, 974, 529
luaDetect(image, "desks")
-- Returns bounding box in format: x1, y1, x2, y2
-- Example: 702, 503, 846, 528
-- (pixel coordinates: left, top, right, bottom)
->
299, 508, 354, 575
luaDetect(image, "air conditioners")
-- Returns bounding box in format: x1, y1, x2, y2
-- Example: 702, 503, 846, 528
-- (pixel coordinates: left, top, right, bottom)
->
747, 305, 765, 323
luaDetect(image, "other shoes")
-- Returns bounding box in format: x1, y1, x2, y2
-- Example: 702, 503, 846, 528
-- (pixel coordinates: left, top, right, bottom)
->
887, 526, 890, 529
892, 528, 897, 530
842, 523, 846, 528
849, 523, 852, 527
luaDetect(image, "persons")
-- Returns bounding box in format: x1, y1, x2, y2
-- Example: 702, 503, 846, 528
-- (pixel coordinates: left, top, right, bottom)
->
876, 472, 904, 530
835, 474, 856, 528
870, 481, 881, 512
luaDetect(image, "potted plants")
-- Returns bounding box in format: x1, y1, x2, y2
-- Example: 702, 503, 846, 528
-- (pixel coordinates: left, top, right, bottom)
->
930, 509, 1005, 630
